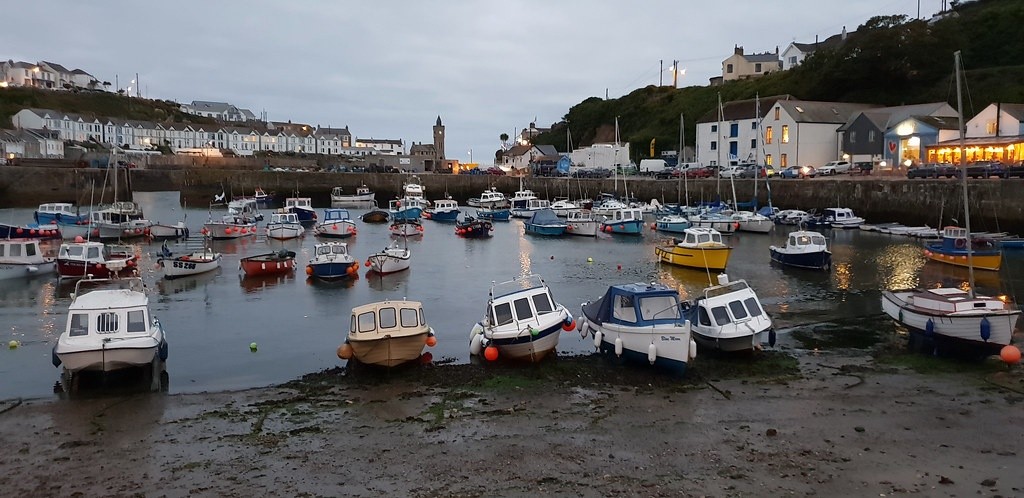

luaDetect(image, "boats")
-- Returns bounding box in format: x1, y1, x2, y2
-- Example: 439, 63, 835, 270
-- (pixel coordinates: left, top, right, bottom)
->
304, 240, 356, 284
239, 247, 297, 279
0, 222, 65, 266
156, 225, 224, 281
146, 220, 189, 242
453, 210, 493, 238
56, 236, 138, 286
49, 177, 169, 396
676, 243, 777, 359
313, 207, 358, 239
365, 188, 412, 276
203, 200, 259, 241
34, 201, 91, 226
334, 296, 437, 375
654, 224, 734, 273
768, 206, 833, 274
266, 211, 305, 242
467, 273, 577, 367
577, 280, 698, 384
202, 115, 1024, 271
523, 208, 568, 237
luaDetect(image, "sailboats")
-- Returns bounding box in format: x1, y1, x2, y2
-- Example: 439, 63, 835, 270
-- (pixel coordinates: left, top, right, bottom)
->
878, 47, 1024, 363
89, 135, 155, 244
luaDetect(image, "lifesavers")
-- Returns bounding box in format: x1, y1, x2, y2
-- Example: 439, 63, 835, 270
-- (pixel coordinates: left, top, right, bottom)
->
955, 238, 965, 249
